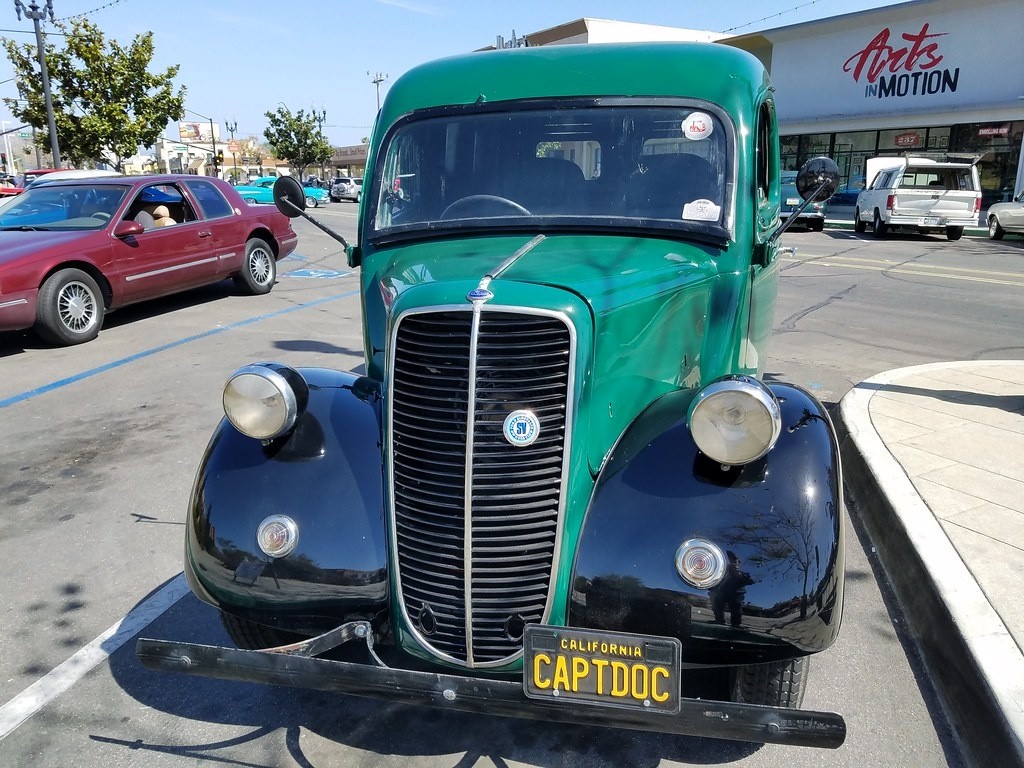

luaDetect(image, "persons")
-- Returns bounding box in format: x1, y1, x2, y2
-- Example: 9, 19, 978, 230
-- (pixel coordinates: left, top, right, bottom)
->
110, 196, 154, 231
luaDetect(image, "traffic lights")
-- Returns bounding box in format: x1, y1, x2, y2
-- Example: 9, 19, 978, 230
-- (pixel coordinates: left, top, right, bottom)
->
217, 148, 225, 163
215, 156, 221, 166
1, 153, 6, 165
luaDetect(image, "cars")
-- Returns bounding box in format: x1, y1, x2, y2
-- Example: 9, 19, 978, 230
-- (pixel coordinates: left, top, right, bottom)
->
0, 168, 123, 217
985, 189, 1024, 241
135, 41, 846, 748
779, 169, 827, 232
141, 186, 182, 202
0, 174, 298, 347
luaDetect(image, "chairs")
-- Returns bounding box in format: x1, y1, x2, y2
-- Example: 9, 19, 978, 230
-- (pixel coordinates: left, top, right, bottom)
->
142, 205, 177, 227
606, 138, 717, 217
490, 157, 590, 216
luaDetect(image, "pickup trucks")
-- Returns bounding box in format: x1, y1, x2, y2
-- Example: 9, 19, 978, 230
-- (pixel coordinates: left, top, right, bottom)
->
235, 176, 331, 208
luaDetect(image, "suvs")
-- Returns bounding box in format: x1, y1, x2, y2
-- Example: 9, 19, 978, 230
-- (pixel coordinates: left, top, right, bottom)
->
331, 178, 364, 203
853, 158, 983, 240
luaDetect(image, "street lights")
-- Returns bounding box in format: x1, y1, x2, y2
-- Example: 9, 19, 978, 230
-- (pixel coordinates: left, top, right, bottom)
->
224, 121, 237, 181
366, 71, 389, 111
15, 0, 62, 169
1, 120, 17, 176
